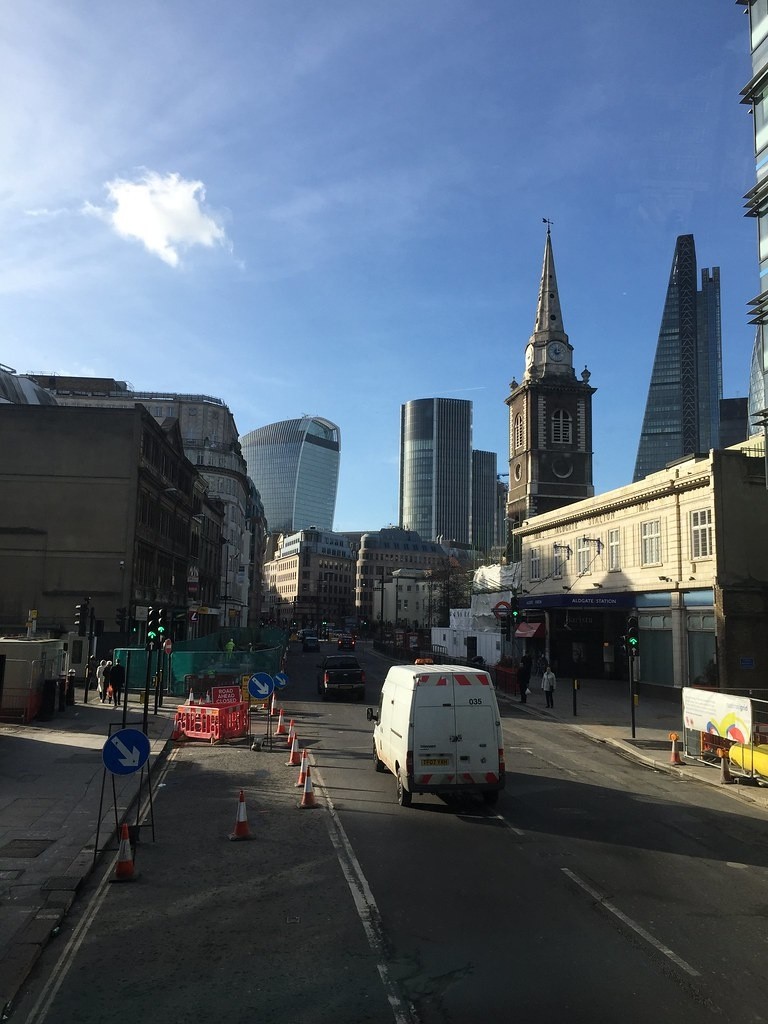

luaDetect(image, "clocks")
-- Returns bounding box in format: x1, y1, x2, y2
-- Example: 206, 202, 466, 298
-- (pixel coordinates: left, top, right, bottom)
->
548, 342, 567, 362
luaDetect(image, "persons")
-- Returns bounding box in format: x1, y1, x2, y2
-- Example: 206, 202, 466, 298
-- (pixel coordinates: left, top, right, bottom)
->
110, 658, 125, 706
97, 660, 106, 702
226, 638, 235, 659
517, 660, 530, 704
521, 650, 532, 668
541, 666, 556, 708
537, 652, 548, 679
102, 661, 112, 704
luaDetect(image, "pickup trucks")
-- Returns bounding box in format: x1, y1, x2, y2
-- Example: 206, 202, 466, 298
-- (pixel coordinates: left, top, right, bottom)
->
315, 654, 367, 702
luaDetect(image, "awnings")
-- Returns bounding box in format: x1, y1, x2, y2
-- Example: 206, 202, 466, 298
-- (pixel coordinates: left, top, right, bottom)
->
512, 554, 634, 610
514, 623, 545, 637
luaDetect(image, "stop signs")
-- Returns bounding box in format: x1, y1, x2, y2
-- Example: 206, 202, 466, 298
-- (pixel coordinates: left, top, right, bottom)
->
163, 638, 173, 654
145, 604, 160, 651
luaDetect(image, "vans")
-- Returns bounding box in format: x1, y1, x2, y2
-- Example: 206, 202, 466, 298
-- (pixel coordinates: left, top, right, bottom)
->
365, 656, 507, 807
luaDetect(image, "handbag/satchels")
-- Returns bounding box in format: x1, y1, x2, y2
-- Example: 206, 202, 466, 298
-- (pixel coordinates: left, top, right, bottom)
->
106, 685, 113, 697
525, 688, 531, 695
550, 685, 554, 692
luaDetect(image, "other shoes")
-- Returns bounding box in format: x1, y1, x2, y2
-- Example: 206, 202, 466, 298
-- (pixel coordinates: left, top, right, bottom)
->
118, 702, 120, 705
109, 701, 111, 704
114, 703, 117, 706
100, 699, 102, 703
546, 705, 550, 708
520, 701, 527, 703
551, 706, 553, 708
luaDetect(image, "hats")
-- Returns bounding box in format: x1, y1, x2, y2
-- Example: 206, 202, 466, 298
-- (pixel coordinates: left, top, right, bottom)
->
100, 660, 106, 665
106, 661, 112, 665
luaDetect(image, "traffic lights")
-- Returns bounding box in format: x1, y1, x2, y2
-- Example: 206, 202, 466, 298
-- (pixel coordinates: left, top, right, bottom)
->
73, 604, 89, 637
128, 616, 138, 634
510, 595, 519, 619
621, 635, 630, 653
501, 615, 511, 643
626, 616, 639, 654
116, 607, 127, 635
156, 608, 168, 648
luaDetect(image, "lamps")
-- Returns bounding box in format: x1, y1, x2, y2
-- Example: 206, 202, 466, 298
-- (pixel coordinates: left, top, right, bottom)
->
659, 576, 668, 582
593, 583, 602, 588
563, 586, 571, 590
523, 590, 529, 594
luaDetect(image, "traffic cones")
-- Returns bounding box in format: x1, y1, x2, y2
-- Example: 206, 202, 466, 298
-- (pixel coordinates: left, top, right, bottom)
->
284, 732, 302, 766
271, 692, 277, 715
669, 736, 687, 768
295, 765, 321, 809
281, 718, 294, 750
227, 789, 256, 841
717, 747, 734, 785
272, 708, 290, 735
105, 822, 141, 883
259, 696, 271, 710
292, 748, 318, 787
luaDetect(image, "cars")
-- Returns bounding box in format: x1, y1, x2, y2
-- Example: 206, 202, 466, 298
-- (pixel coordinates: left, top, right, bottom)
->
294, 627, 358, 652
